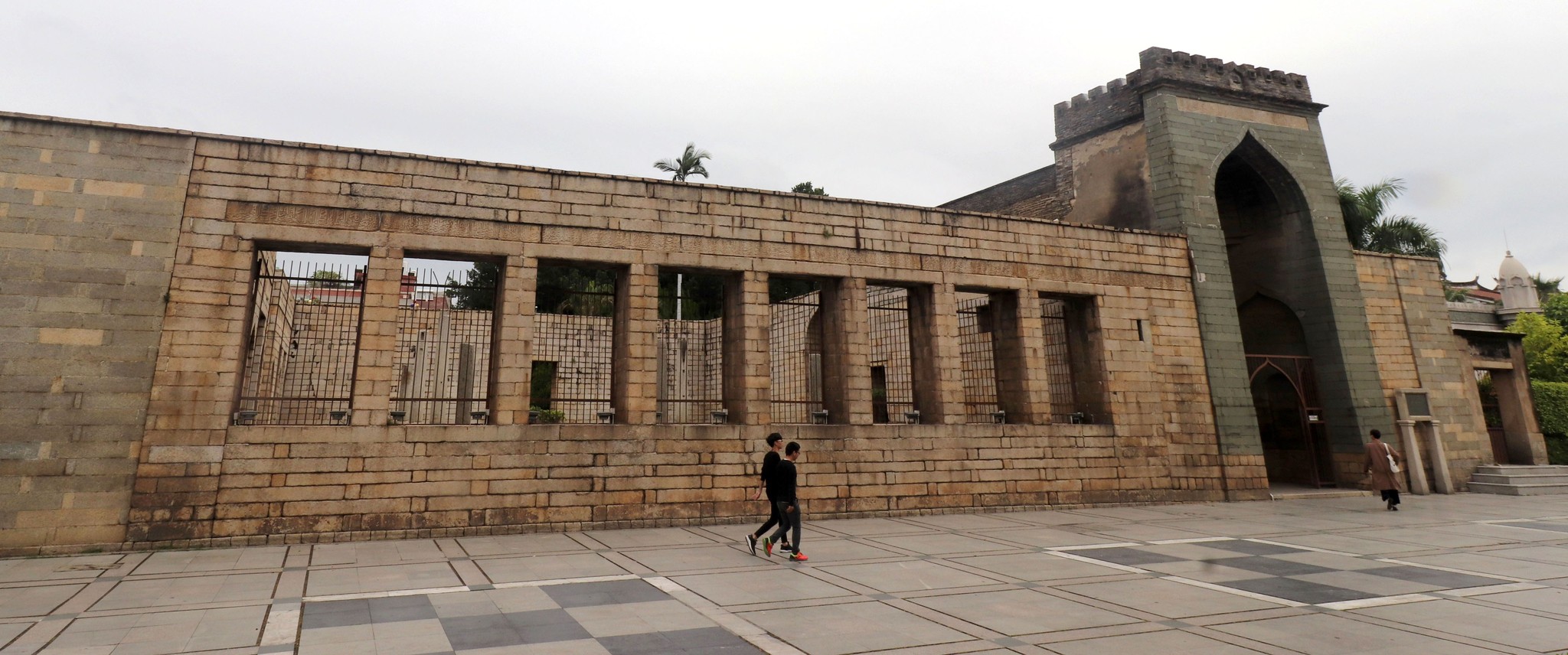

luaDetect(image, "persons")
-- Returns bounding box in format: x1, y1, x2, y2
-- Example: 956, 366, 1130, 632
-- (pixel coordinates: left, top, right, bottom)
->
745, 433, 800, 555
762, 442, 808, 562
1364, 429, 1401, 511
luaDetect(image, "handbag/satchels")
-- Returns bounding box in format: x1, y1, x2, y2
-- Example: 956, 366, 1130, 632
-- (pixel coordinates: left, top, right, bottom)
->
1384, 442, 1400, 473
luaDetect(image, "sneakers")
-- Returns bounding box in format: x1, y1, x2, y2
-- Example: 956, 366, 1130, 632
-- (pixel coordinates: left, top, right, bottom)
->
780, 541, 793, 552
746, 534, 757, 555
789, 551, 808, 562
762, 538, 774, 557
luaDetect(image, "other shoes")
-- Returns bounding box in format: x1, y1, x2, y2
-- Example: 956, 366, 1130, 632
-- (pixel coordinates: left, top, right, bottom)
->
1387, 498, 1393, 510
1392, 506, 1398, 511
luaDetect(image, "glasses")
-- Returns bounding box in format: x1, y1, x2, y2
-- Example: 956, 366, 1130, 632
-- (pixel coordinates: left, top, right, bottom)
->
797, 452, 800, 455
777, 440, 782, 443
